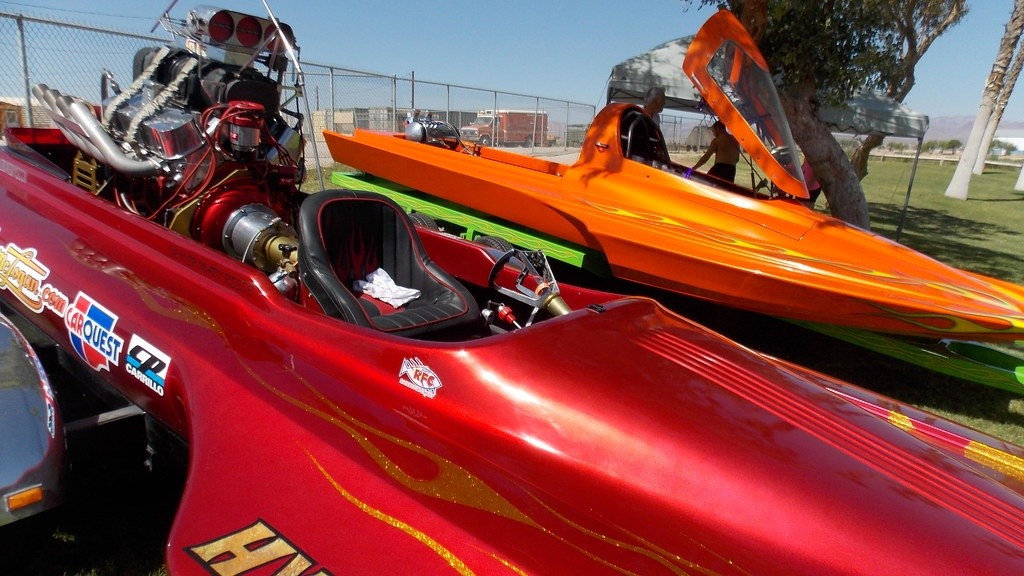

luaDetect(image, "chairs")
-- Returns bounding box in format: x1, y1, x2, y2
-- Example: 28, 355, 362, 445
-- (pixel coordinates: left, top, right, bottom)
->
306, 188, 482, 339
623, 112, 670, 166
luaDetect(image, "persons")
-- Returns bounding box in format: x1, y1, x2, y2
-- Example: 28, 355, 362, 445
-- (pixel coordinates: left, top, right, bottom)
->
796, 156, 821, 202
691, 121, 741, 183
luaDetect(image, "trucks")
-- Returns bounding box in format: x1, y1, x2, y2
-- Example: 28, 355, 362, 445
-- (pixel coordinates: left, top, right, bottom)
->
458, 109, 548, 148
